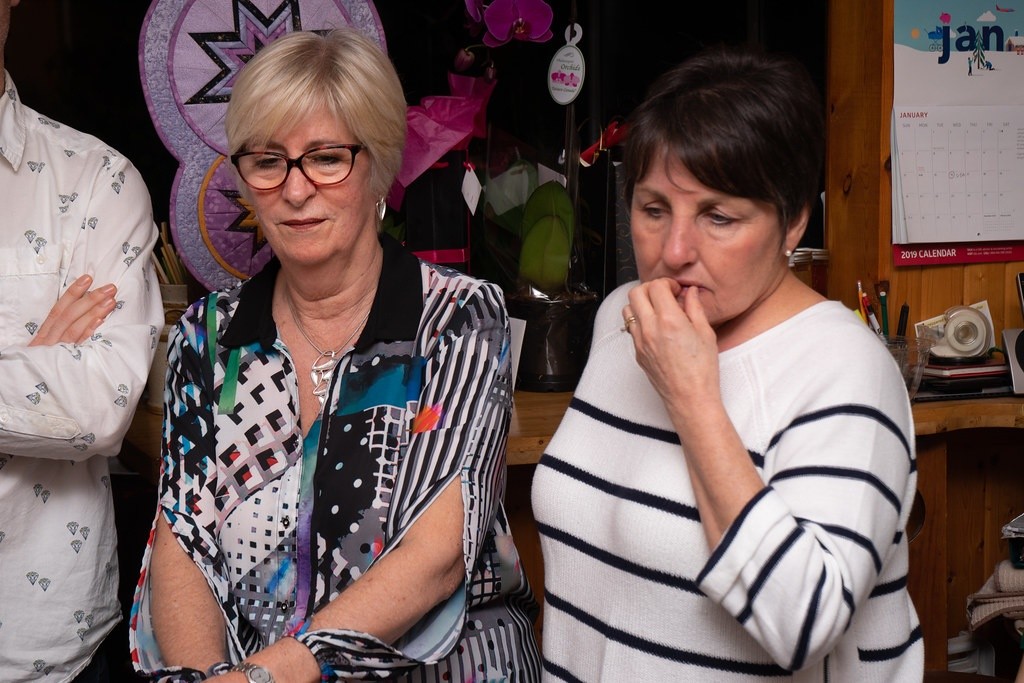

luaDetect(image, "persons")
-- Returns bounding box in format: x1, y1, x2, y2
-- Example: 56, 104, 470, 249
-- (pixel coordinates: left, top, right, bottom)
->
529, 55, 925, 682
1, 1, 163, 682
126, 28, 540, 683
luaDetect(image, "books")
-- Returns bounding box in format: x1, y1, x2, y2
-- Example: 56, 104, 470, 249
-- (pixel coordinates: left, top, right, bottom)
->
923, 363, 1011, 379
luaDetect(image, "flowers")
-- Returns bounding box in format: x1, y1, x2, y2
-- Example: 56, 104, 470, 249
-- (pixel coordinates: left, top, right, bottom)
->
391, 0, 635, 302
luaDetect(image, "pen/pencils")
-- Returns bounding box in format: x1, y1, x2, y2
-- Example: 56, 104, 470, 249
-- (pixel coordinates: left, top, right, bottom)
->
851, 278, 911, 342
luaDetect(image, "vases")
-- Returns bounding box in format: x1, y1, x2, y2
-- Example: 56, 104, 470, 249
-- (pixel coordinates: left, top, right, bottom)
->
472, 48, 614, 393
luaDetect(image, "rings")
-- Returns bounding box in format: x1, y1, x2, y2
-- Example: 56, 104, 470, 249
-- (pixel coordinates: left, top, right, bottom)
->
623, 316, 638, 334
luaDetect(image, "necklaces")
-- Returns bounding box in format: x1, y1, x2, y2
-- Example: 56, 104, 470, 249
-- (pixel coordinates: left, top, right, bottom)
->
284, 276, 372, 408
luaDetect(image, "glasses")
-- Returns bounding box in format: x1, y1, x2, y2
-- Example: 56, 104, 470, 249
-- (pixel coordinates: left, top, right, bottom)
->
229, 143, 364, 191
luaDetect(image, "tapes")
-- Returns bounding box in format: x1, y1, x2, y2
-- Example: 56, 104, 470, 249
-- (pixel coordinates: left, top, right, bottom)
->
930, 304, 992, 359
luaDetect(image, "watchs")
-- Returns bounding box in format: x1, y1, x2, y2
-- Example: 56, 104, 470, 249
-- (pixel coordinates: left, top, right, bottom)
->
227, 662, 275, 683
